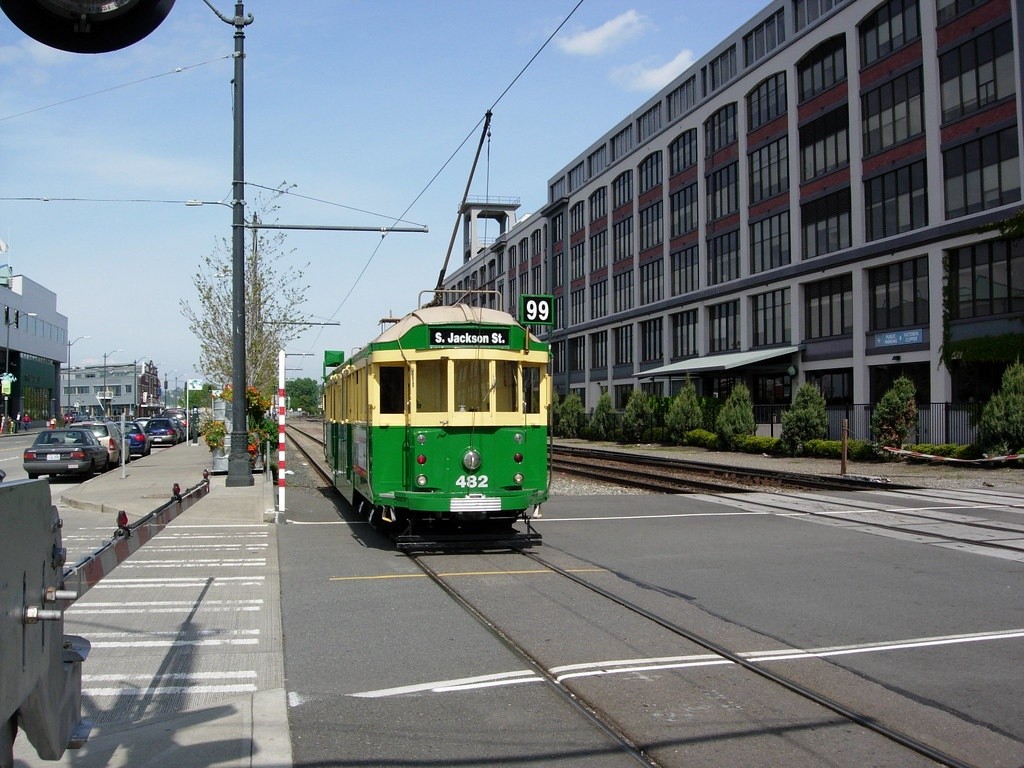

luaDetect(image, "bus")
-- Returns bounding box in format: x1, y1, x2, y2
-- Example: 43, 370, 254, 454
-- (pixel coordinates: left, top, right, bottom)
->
319, 110, 555, 555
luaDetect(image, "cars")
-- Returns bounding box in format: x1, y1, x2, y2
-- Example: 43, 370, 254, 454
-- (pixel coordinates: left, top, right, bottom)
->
22, 427, 109, 480
62, 410, 89, 423
115, 421, 151, 456
133, 409, 193, 445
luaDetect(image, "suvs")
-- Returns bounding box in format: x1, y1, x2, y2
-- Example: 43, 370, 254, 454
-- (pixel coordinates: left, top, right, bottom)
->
64, 419, 131, 467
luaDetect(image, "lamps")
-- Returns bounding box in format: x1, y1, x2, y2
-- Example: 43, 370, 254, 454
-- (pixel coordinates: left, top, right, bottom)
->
892, 356, 900, 361
787, 366, 798, 378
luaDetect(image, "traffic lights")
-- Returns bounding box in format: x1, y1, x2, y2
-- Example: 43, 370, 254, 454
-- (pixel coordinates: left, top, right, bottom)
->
208, 385, 212, 393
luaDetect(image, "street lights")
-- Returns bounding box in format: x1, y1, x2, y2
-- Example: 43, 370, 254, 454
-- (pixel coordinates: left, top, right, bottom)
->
102, 349, 124, 422
184, 198, 259, 387
164, 369, 178, 409
134, 355, 152, 420
4, 313, 38, 434
67, 336, 92, 428
175, 374, 187, 409
149, 362, 167, 418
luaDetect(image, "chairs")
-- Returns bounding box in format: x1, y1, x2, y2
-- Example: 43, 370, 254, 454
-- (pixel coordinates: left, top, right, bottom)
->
49, 438, 58, 443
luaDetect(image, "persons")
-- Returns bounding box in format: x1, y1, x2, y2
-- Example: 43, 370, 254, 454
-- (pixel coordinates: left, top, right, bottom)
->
0, 412, 20, 434
23, 414, 31, 432
